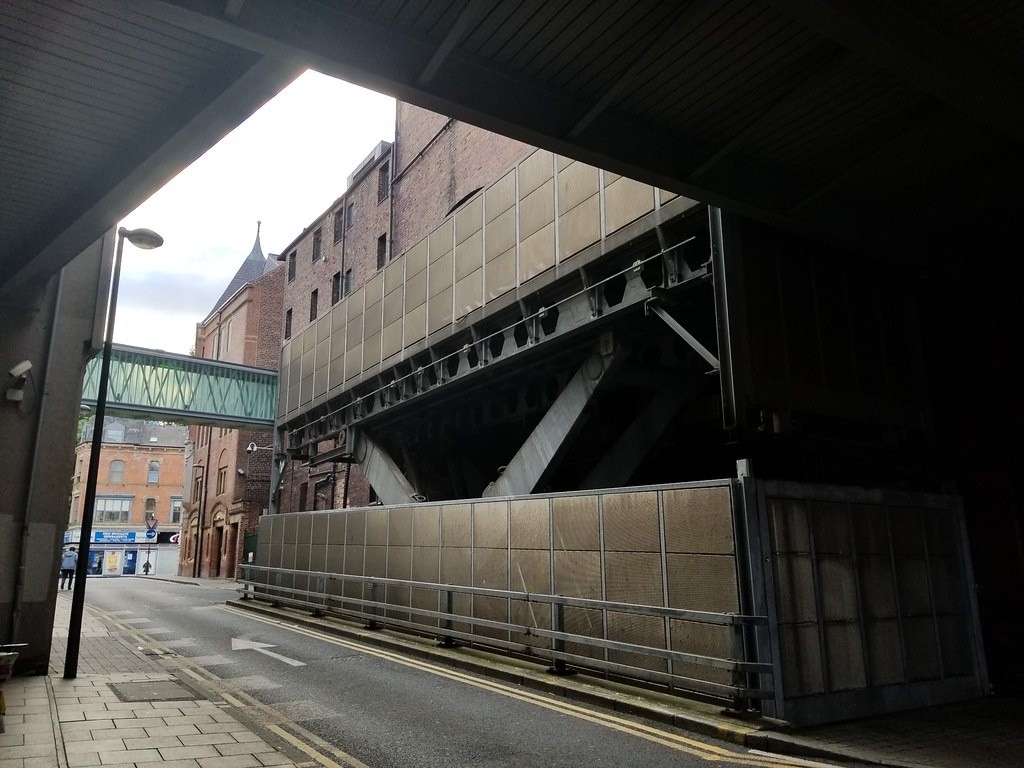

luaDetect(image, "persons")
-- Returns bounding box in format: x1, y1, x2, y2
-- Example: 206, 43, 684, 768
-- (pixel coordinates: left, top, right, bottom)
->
60, 546, 78, 591
96, 557, 102, 575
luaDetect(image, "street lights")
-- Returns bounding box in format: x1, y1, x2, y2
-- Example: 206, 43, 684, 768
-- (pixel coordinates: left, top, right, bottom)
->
63, 225, 165, 679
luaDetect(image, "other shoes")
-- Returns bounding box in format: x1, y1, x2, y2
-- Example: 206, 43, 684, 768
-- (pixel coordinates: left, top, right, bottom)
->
68, 587, 71, 590
61, 582, 64, 589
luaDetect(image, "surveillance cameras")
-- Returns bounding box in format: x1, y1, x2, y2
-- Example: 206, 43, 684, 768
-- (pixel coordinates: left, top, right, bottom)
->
238, 469, 244, 474
247, 447, 252, 454
8, 360, 32, 377
